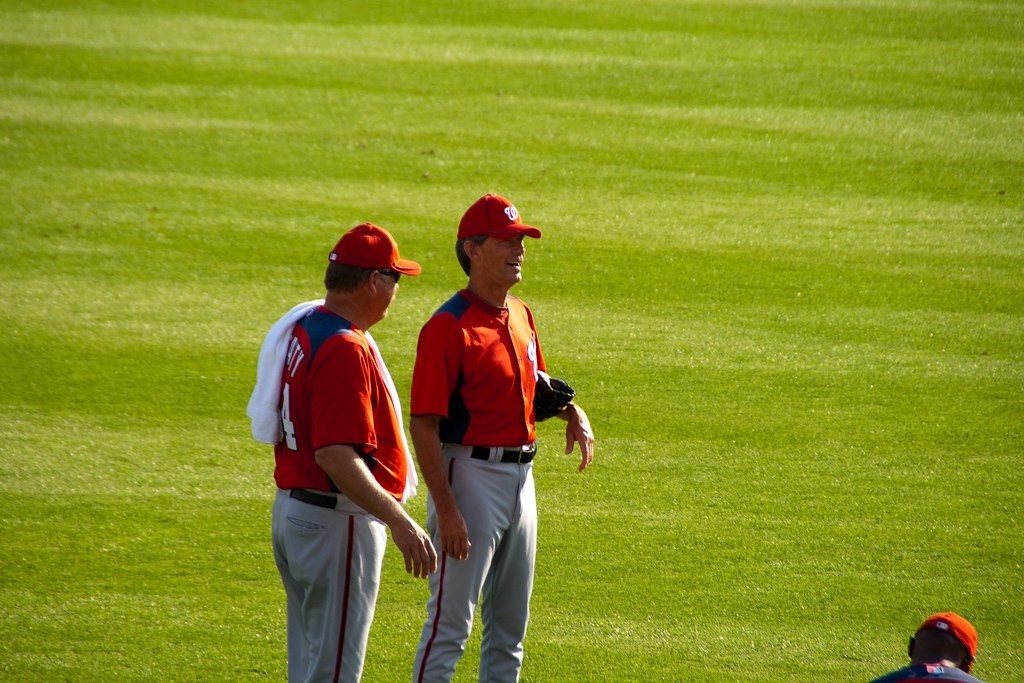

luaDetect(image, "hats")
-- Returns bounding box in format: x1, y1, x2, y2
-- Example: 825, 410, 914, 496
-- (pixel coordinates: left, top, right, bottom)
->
915, 611, 978, 674
328, 222, 422, 276
457, 193, 541, 240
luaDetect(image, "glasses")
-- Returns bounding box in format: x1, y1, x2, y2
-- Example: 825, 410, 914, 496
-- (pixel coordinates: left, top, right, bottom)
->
355, 270, 401, 283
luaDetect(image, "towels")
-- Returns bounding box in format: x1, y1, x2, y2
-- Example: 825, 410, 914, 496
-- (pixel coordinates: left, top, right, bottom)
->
247, 299, 419, 505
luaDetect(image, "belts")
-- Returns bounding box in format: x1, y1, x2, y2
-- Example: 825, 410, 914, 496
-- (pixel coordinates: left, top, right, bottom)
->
290, 487, 337, 510
440, 441, 538, 465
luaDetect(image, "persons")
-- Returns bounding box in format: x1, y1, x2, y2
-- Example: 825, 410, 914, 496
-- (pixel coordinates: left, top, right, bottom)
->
409, 194, 595, 683
871, 612, 982, 683
271, 222, 437, 683
